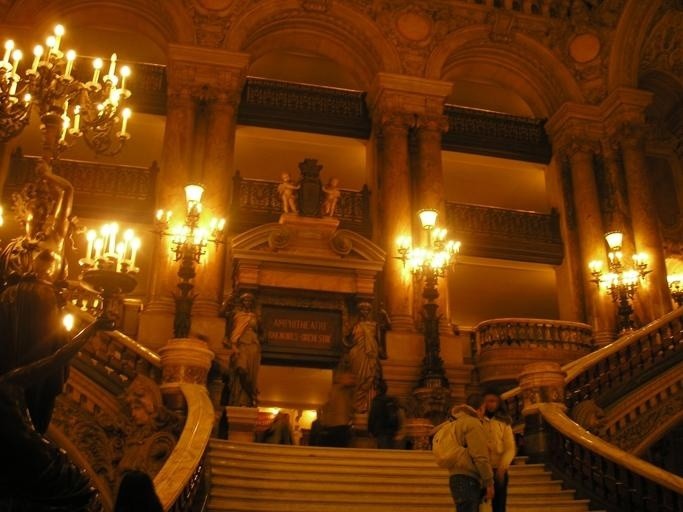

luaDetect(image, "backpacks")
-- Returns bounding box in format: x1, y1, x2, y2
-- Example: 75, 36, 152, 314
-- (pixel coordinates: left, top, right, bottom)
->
431, 413, 471, 468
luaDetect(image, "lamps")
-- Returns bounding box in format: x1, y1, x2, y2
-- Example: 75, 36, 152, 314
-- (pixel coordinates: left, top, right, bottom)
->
585, 226, 683, 332
392, 209, 464, 382
154, 183, 228, 263
2, 23, 136, 156
77, 220, 142, 294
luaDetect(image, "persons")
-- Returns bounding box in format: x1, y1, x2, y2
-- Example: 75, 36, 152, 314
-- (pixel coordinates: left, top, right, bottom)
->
1, 272, 104, 512
321, 176, 340, 218
480, 390, 517, 512
342, 302, 392, 413
449, 393, 495, 512
112, 470, 162, 512
218, 292, 264, 408
261, 383, 353, 447
278, 173, 301, 213
369, 380, 401, 448
